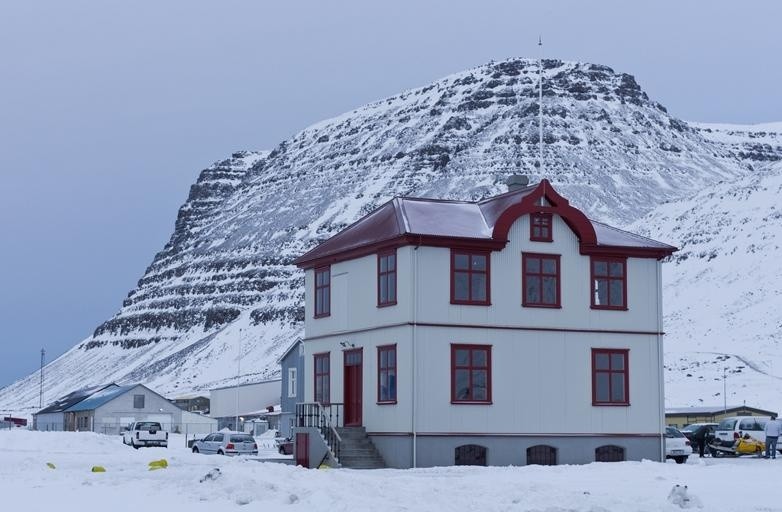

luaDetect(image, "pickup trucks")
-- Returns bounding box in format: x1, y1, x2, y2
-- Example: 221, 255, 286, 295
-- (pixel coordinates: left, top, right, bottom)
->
120, 420, 168, 449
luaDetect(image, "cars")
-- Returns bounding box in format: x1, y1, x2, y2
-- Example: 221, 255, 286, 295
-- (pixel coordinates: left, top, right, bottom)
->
278, 434, 294, 456
665, 422, 721, 464
191, 431, 258, 457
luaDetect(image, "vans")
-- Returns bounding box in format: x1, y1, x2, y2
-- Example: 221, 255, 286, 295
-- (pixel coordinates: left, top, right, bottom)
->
714, 415, 782, 454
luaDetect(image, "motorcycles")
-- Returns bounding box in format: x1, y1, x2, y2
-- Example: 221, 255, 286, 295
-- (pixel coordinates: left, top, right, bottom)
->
706, 431, 765, 459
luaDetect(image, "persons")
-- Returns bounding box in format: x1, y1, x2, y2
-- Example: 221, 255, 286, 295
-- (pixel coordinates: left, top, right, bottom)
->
694, 424, 713, 458
762, 415, 782, 459
